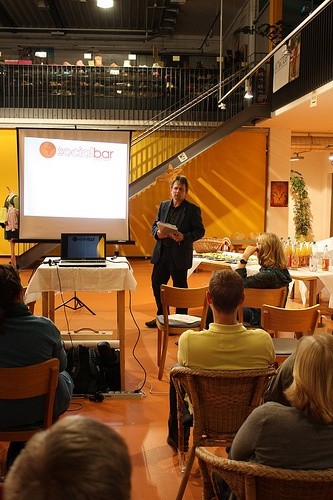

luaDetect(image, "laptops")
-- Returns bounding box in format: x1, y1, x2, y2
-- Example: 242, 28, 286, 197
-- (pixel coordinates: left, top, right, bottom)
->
58, 232, 107, 267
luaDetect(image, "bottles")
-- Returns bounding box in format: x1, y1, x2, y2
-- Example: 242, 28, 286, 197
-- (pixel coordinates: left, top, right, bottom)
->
321, 245, 329, 271
290, 237, 301, 270
280, 237, 292, 269
301, 241, 318, 272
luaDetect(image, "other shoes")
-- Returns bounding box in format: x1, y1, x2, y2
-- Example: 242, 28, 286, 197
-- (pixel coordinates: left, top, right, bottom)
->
167, 434, 188, 452
145, 319, 157, 328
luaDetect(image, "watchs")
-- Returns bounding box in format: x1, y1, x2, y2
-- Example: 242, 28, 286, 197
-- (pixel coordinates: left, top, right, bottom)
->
240, 259, 248, 264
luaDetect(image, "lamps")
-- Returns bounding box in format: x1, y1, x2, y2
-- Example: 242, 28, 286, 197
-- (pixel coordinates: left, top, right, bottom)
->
290, 152, 304, 162
243, 78, 254, 99
208, 29, 214, 38
205, 41, 209, 47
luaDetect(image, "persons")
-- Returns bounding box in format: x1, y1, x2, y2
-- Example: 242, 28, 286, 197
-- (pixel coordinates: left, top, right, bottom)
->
3, 193, 19, 264
0, 262, 74, 471
144, 174, 205, 334
205, 233, 293, 328
0, 415, 133, 500
178, 269, 333, 500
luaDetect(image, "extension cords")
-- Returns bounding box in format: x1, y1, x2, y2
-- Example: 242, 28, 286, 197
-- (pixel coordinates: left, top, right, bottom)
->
89, 390, 145, 399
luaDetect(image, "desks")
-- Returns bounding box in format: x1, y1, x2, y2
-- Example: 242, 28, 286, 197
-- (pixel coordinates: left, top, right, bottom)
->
186, 249, 333, 310
24, 257, 137, 390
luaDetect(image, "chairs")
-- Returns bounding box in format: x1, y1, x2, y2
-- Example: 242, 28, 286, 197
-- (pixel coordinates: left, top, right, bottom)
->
237, 286, 288, 338
195, 445, 333, 500
169, 365, 279, 500
22, 286, 36, 315
155, 284, 210, 380
0, 358, 61, 442
261, 303, 322, 368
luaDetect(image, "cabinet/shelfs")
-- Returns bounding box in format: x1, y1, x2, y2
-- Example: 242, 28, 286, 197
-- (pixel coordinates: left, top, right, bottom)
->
0, 66, 231, 110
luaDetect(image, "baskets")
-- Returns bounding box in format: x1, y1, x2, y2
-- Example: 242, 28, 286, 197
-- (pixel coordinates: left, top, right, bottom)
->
194, 237, 224, 253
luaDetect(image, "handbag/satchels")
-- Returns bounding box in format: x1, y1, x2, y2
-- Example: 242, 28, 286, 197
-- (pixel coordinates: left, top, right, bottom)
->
68, 341, 121, 394
